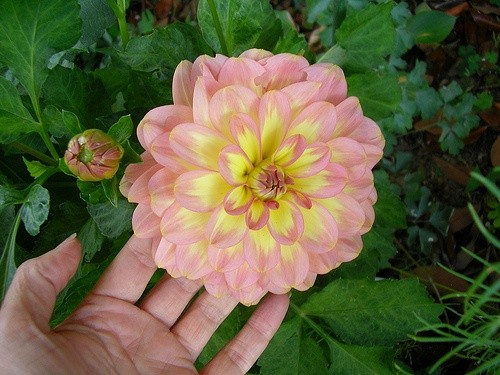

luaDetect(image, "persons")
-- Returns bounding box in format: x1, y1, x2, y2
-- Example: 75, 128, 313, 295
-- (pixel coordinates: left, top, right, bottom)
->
0, 230, 295, 375
